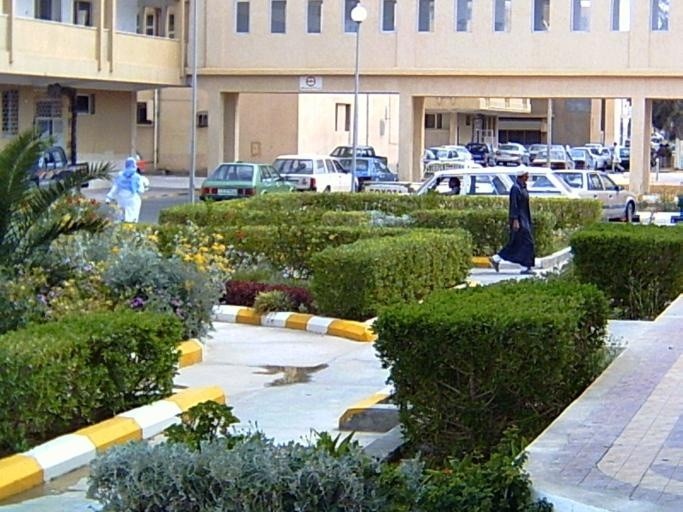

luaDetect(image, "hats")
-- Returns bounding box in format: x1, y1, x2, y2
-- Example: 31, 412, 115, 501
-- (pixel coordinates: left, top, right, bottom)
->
516, 164, 528, 176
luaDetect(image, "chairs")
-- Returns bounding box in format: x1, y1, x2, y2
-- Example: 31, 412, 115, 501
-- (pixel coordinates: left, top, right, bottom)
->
294, 164, 305, 173
493, 178, 504, 195
228, 171, 237, 179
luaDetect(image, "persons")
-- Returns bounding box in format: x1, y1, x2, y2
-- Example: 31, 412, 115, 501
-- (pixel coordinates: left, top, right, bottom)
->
670, 181, 683, 223
444, 177, 460, 195
655, 144, 672, 159
105, 157, 150, 223
607, 142, 625, 174
488, 163, 538, 274
136, 152, 143, 176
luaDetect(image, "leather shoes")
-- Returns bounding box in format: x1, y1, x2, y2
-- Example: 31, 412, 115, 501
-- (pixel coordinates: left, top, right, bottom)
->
488, 256, 499, 272
521, 269, 535, 273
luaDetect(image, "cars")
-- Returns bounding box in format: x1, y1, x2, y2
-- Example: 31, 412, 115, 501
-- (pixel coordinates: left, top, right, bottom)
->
327, 140, 637, 220
261, 154, 359, 193
199, 160, 297, 201
529, 169, 637, 223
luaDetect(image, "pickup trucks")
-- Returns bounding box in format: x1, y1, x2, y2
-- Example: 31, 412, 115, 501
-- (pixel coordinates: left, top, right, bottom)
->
29, 144, 90, 191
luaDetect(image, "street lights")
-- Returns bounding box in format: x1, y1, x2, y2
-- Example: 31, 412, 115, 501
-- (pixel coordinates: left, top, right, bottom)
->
350, 2, 368, 193
